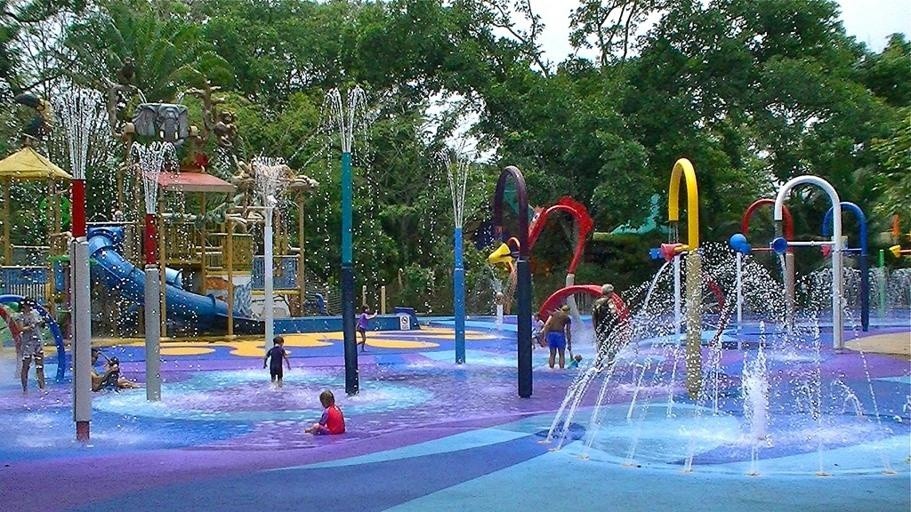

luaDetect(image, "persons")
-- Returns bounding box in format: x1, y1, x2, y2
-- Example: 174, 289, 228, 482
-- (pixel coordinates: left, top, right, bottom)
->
567, 349, 583, 369
304, 390, 347, 436
355, 304, 378, 353
15, 298, 44, 392
591, 283, 622, 378
90, 349, 139, 392
541, 304, 573, 370
263, 337, 290, 385
531, 312, 544, 349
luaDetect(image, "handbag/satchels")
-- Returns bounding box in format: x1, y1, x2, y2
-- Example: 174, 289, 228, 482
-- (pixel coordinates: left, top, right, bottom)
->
597, 304, 618, 325
